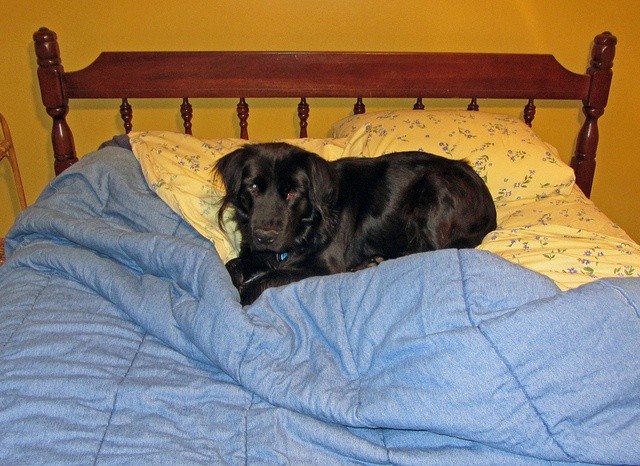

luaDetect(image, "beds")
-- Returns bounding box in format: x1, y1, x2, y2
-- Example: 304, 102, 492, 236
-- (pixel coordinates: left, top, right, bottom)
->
1, 28, 640, 466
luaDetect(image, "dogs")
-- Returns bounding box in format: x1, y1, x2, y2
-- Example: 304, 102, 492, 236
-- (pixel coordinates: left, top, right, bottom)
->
210, 139, 500, 307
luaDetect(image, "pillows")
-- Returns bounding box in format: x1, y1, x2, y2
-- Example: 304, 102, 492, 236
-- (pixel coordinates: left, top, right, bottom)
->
128, 129, 350, 264
330, 109, 576, 209
